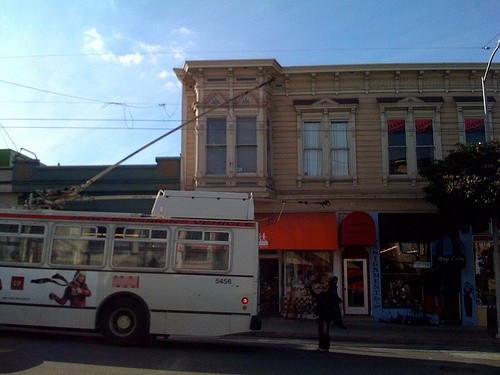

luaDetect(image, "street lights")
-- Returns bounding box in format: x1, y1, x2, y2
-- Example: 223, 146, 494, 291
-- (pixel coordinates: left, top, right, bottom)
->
481, 38, 500, 339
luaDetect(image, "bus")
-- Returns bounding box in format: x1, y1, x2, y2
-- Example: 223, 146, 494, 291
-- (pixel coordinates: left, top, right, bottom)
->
0, 190, 261, 347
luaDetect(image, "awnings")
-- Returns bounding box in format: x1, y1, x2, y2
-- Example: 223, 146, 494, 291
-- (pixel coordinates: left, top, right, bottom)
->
255, 212, 338, 250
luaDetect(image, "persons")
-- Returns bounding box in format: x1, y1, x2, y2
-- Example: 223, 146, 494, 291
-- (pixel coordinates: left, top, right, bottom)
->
461, 281, 474, 317
309, 276, 346, 352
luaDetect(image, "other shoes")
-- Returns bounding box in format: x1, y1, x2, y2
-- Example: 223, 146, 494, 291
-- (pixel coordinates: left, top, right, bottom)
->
338, 325, 348, 329
314, 349, 328, 353
